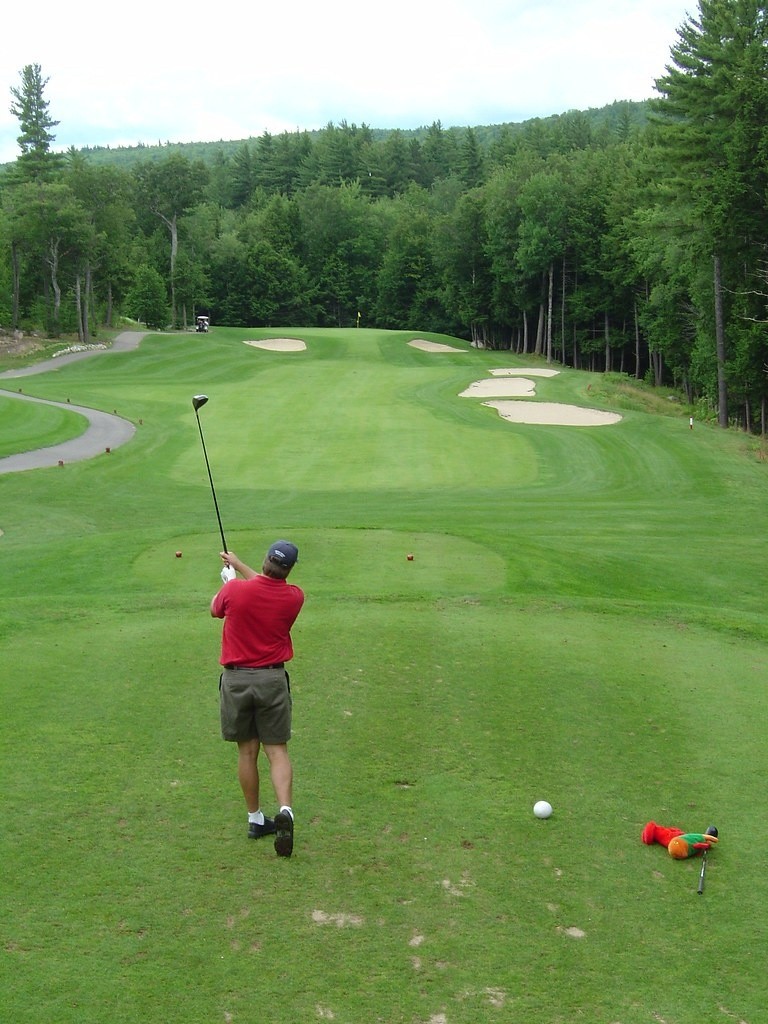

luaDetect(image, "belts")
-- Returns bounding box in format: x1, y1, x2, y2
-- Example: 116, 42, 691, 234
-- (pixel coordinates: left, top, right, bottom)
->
224, 664, 284, 670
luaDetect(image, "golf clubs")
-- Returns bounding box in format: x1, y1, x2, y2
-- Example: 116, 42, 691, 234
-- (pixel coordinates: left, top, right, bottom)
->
192, 394, 231, 570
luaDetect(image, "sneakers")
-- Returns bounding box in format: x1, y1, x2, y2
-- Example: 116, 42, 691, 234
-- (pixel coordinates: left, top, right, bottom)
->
274, 810, 293, 857
247, 816, 276, 840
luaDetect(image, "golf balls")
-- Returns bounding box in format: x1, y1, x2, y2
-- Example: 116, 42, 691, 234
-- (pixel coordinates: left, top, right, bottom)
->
533, 800, 553, 819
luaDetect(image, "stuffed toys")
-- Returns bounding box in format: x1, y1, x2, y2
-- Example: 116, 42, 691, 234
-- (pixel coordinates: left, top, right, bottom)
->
641, 821, 719, 860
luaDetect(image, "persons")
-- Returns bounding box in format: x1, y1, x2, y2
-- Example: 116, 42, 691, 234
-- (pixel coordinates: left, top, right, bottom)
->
210, 540, 304, 858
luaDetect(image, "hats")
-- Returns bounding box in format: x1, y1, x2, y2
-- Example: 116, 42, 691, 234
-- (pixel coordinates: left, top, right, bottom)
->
268, 540, 299, 567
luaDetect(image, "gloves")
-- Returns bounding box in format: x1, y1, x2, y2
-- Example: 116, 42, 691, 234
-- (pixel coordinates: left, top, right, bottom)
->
220, 565, 236, 584
